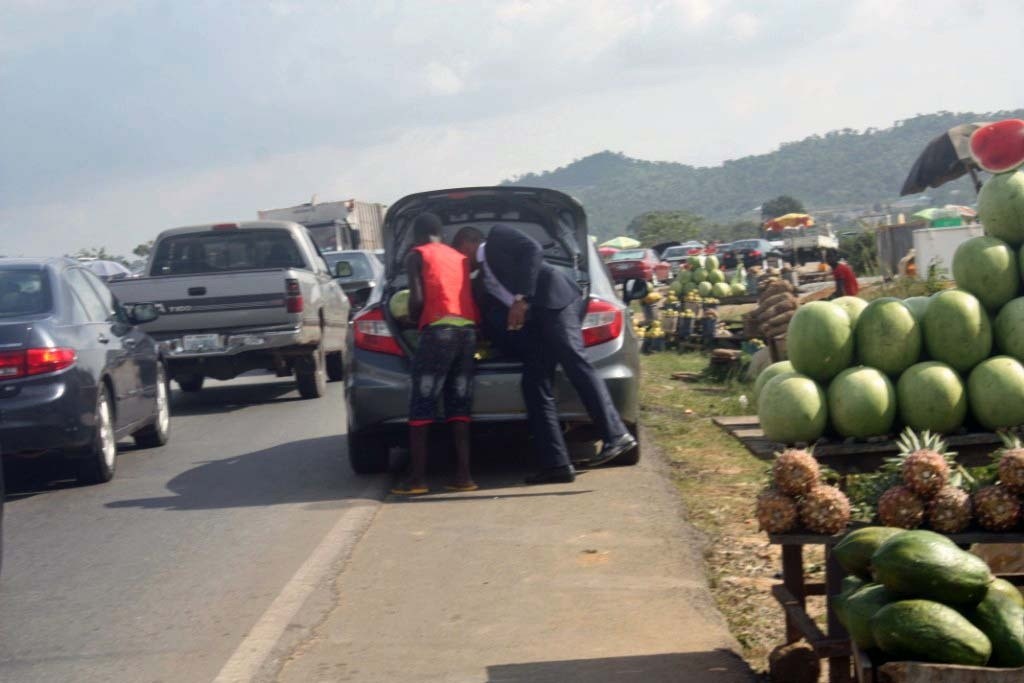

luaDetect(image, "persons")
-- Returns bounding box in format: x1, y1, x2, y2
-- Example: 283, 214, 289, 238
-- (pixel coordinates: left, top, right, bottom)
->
454, 224, 639, 484
821, 257, 859, 300
390, 213, 479, 495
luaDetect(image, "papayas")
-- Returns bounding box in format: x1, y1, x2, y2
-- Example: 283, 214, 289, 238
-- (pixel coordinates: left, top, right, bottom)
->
828, 525, 1024, 671
632, 288, 700, 338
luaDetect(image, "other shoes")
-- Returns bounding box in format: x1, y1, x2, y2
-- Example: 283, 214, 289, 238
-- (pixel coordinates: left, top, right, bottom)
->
524, 463, 575, 485
581, 433, 638, 467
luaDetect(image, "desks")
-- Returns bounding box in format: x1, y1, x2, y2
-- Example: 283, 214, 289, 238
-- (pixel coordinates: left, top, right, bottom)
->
700, 293, 765, 307
709, 414, 1005, 464
801, 271, 830, 282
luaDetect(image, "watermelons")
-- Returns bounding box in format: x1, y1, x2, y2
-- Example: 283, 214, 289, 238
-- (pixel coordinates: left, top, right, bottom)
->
670, 255, 746, 298
757, 118, 1024, 437
389, 289, 411, 317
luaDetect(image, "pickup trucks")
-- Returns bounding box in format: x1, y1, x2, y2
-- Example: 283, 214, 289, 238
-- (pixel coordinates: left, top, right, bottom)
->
104, 220, 353, 398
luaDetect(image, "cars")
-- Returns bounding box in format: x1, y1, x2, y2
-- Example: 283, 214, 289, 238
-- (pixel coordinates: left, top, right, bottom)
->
605, 238, 785, 287
0, 258, 170, 482
325, 246, 387, 312
342, 183, 645, 476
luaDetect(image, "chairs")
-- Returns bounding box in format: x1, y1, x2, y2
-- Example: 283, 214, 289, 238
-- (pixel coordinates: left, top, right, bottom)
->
187, 245, 212, 271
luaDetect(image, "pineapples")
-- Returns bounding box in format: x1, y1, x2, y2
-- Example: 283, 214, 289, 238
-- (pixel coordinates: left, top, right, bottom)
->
753, 426, 1024, 535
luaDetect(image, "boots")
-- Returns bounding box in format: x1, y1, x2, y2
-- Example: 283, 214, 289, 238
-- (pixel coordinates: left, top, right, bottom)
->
389, 423, 431, 495
439, 420, 479, 491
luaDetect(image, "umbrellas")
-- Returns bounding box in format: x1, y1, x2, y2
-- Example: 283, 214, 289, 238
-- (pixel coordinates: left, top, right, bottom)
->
597, 237, 641, 256
762, 211, 816, 265
83, 261, 129, 276
899, 122, 991, 197
912, 208, 941, 220
